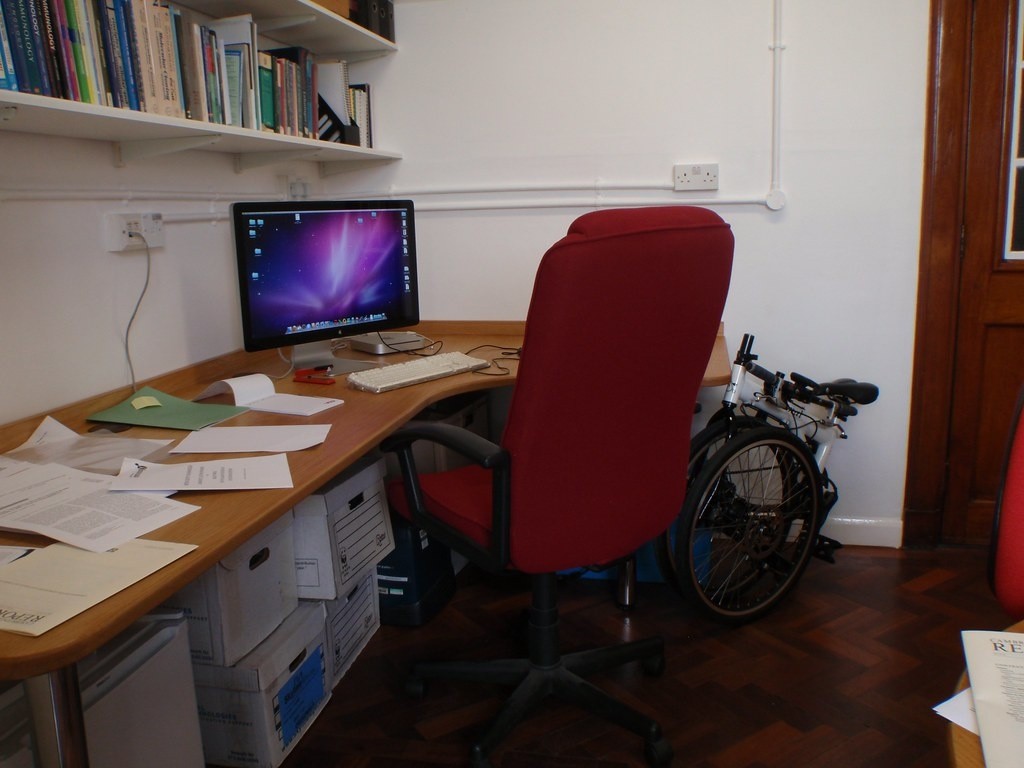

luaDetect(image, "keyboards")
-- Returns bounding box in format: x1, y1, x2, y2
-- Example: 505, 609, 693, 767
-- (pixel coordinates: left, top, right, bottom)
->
347, 350, 489, 395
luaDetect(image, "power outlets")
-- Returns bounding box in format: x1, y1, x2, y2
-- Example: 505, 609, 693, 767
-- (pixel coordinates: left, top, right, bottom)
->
141, 210, 167, 250
672, 164, 719, 192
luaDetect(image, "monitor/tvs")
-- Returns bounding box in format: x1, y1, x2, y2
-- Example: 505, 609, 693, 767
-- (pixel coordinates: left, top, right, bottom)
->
228, 200, 419, 351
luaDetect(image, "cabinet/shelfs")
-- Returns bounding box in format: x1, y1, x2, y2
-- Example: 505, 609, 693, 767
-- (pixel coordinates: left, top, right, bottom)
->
0, 0, 407, 164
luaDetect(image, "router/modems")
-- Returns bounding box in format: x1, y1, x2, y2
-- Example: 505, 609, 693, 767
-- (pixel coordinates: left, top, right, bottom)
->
350, 332, 424, 355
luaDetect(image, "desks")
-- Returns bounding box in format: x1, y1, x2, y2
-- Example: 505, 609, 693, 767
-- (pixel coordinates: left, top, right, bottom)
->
0, 319, 734, 768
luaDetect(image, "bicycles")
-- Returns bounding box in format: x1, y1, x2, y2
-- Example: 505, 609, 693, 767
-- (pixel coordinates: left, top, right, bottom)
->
656, 334, 880, 619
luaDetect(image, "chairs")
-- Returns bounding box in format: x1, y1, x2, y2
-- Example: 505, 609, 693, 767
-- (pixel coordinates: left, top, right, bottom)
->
373, 204, 739, 767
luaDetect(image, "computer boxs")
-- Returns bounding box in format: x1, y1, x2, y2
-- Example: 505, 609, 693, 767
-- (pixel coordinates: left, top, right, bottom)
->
380, 491, 458, 630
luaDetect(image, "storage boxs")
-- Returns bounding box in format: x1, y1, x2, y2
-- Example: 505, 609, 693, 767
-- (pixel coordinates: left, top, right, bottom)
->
184, 599, 335, 768
378, 514, 458, 632
287, 450, 399, 601
148, 503, 300, 669
321, 563, 380, 696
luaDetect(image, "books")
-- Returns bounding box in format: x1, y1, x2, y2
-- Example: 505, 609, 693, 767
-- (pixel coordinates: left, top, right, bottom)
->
0, 538, 201, 637
961, 630, 1024, 768
84, 384, 251, 431
0, 0, 371, 150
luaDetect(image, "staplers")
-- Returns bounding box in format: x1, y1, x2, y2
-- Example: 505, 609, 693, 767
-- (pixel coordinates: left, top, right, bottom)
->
292, 363, 336, 386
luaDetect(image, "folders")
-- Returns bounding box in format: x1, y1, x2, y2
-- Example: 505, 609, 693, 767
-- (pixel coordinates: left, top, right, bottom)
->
349, 0, 395, 42
